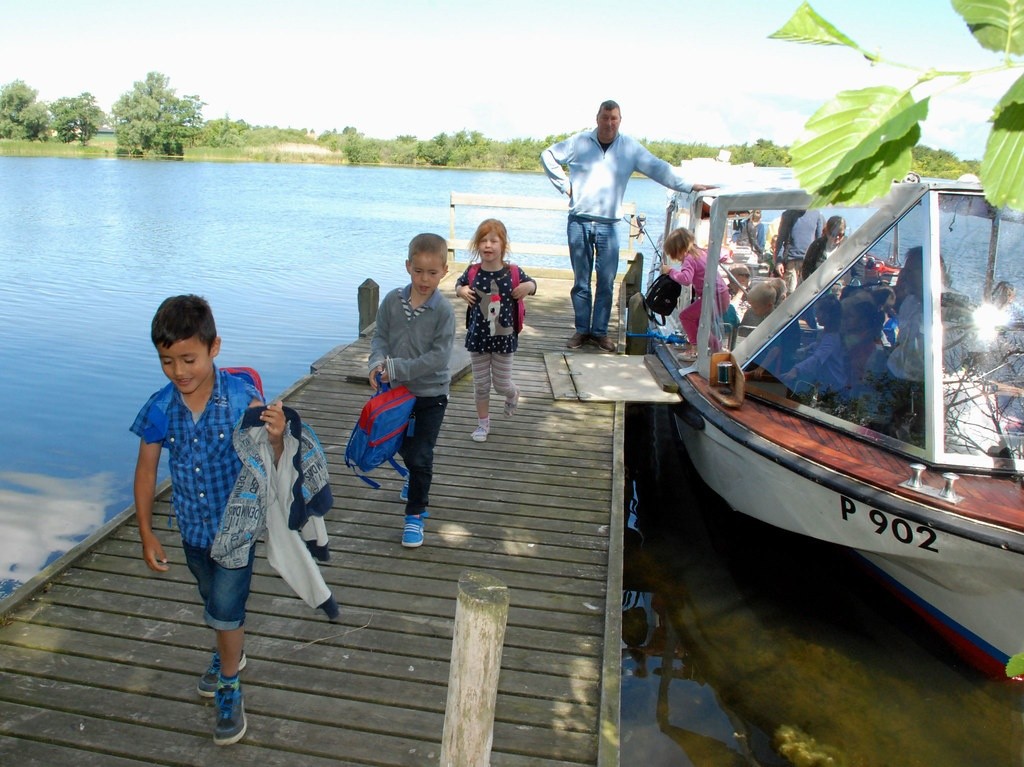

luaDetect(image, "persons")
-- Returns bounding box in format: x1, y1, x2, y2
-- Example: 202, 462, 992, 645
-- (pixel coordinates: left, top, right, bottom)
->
128, 294, 286, 746
865, 245, 974, 447
731, 210, 781, 270
455, 219, 537, 443
541, 100, 720, 352
722, 263, 899, 435
659, 227, 730, 361
368, 233, 456, 548
992, 280, 1024, 318
774, 208, 827, 293
801, 215, 864, 298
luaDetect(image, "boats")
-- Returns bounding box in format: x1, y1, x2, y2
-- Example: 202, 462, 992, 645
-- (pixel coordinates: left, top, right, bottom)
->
641, 148, 1024, 687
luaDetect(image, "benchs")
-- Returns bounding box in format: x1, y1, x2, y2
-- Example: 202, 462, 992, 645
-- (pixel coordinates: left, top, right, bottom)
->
729, 245, 820, 328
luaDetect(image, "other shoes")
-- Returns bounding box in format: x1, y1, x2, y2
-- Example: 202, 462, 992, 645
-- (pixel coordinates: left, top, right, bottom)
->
471, 418, 490, 442
676, 349, 697, 361
503, 389, 519, 418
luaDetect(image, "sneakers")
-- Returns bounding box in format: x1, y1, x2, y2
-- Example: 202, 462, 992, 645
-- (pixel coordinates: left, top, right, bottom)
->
400, 478, 410, 501
212, 677, 247, 745
196, 647, 247, 698
589, 330, 615, 352
567, 332, 589, 348
402, 514, 425, 548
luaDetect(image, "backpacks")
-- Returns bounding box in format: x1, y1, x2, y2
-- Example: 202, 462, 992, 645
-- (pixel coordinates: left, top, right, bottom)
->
345, 374, 417, 489
466, 264, 524, 333
641, 273, 682, 325
218, 366, 267, 406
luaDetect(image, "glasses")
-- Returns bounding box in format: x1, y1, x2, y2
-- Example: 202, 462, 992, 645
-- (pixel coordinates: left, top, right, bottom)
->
828, 230, 845, 237
